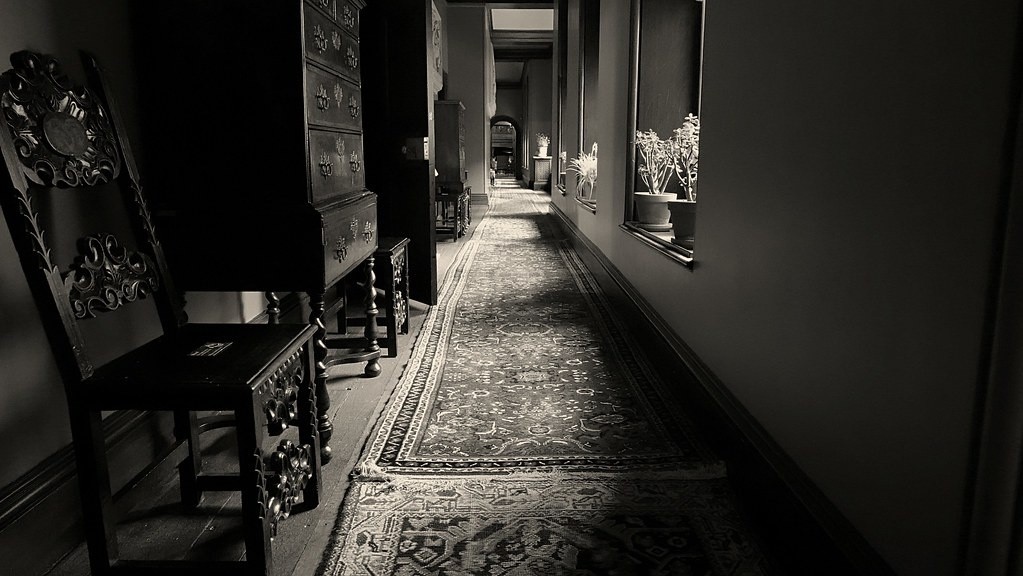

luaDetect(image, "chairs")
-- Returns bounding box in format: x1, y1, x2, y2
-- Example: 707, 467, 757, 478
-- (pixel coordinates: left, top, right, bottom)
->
0, 51, 322, 576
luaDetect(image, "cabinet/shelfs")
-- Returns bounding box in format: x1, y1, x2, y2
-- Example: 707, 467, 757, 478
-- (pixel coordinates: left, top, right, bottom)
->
434, 101, 472, 235
126, 0, 378, 442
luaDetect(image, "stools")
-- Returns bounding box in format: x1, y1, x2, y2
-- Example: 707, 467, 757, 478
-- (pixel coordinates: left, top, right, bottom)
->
435, 193, 464, 242
322, 237, 412, 357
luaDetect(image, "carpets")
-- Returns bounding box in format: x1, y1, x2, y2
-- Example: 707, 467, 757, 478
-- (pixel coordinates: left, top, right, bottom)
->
322, 239, 769, 576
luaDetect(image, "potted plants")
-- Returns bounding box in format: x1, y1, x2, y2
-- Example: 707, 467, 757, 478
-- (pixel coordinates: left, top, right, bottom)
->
629, 113, 700, 248
566, 142, 598, 203
536, 133, 550, 156
